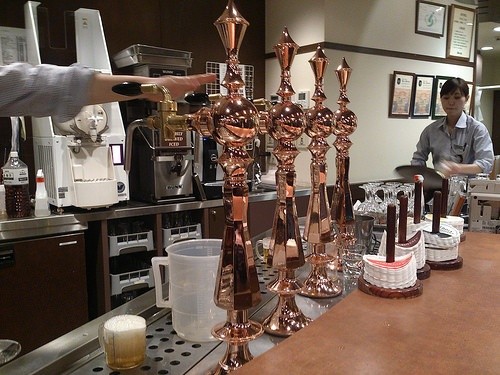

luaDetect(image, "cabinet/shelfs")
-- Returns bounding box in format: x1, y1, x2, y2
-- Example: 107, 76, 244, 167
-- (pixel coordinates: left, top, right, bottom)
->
0, 195, 330, 359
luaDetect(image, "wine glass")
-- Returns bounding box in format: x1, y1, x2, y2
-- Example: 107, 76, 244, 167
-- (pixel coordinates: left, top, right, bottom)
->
357, 182, 415, 224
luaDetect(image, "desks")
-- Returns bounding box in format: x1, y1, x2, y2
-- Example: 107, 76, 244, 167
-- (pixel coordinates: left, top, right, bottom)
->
220, 223, 500, 374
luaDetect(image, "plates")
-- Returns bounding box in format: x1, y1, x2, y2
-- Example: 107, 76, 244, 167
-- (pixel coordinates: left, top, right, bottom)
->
0, 339, 21, 364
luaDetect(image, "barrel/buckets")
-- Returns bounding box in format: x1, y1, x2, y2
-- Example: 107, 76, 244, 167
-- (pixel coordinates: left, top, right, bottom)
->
332, 215, 375, 254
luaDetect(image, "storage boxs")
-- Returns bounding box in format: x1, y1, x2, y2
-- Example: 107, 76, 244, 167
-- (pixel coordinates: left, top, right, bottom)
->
134, 66, 188, 82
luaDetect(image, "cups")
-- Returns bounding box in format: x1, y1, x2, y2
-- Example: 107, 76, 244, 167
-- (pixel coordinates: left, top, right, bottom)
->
339, 243, 367, 279
255, 236, 272, 263
97, 314, 147, 370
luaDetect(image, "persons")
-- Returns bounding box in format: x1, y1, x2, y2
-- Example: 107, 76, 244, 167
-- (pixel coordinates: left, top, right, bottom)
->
411, 78, 494, 213
0, 61, 218, 124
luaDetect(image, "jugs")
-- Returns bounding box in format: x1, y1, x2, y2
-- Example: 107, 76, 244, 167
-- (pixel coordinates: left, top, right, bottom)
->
151, 238, 228, 342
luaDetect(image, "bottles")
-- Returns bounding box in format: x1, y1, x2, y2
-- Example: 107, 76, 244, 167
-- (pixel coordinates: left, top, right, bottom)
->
34, 169, 50, 218
1, 151, 31, 220
